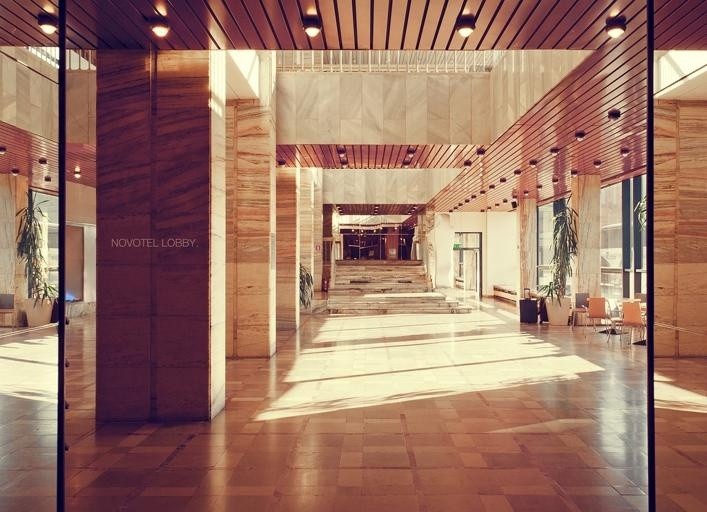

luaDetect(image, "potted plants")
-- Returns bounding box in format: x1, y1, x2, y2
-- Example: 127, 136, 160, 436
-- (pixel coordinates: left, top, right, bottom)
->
12, 186, 58, 328
532, 191, 583, 326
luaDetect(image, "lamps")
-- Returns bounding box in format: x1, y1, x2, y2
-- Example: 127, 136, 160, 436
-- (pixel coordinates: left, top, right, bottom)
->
149, 19, 172, 38
303, 20, 323, 38
456, 19, 476, 37
38, 18, 59, 36
605, 20, 626, 38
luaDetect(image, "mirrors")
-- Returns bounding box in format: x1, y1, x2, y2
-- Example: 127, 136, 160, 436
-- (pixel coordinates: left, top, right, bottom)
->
647, 1, 707, 511
1, 0, 64, 510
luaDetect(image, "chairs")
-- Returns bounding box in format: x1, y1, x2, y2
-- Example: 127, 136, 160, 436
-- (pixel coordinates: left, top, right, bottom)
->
570, 291, 646, 347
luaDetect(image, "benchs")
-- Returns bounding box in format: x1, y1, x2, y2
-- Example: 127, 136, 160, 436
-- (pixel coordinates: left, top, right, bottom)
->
493, 283, 519, 301
